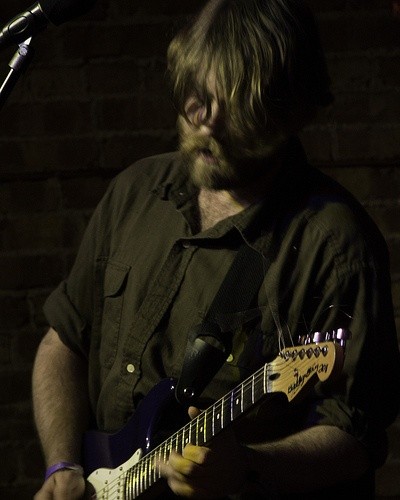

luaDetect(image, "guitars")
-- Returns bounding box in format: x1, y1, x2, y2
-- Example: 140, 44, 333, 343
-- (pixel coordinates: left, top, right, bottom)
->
75, 304, 356, 500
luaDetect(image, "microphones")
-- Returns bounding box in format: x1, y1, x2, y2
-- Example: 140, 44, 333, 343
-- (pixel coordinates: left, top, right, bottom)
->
0, 0, 59, 42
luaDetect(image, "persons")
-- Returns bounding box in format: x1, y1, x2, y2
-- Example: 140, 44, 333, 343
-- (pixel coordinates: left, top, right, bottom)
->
23, 0, 376, 499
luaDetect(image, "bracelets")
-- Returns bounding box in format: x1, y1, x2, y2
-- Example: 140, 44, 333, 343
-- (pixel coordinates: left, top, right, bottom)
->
42, 461, 81, 478
